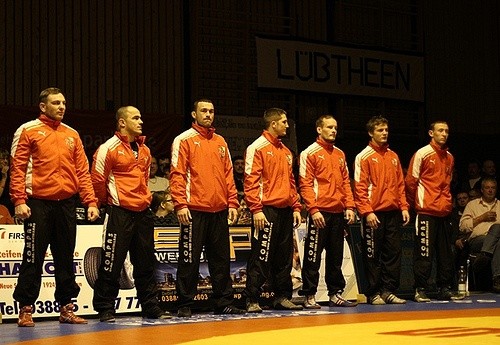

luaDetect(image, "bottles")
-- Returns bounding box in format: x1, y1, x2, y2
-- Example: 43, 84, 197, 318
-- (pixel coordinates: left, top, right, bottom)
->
458, 266, 466, 292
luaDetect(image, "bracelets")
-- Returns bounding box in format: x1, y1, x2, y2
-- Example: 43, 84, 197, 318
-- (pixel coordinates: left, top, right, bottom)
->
0, 181, 5, 188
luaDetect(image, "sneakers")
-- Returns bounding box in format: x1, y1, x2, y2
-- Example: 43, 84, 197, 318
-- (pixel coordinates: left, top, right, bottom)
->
440, 287, 463, 300
245, 301, 262, 312
177, 308, 190, 320
370, 293, 386, 305
382, 291, 406, 304
213, 305, 245, 316
59, 303, 88, 324
304, 295, 321, 308
100, 314, 116, 323
143, 311, 171, 320
493, 275, 500, 292
329, 293, 357, 307
18, 305, 35, 327
473, 252, 490, 268
413, 288, 431, 302
273, 297, 304, 311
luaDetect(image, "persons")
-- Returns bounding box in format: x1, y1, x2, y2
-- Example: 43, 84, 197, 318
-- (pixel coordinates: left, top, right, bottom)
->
405, 120, 465, 302
169, 97, 247, 318
459, 179, 500, 292
298, 114, 358, 309
243, 107, 304, 312
354, 115, 410, 305
0, 134, 500, 256
91, 105, 177, 322
9, 88, 100, 328
